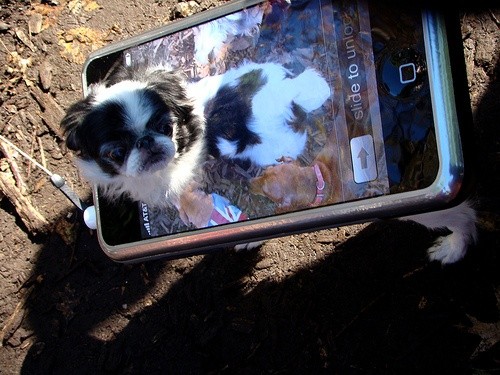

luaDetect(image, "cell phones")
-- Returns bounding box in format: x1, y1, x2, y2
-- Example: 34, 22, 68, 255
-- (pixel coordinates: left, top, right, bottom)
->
81, 0, 475, 264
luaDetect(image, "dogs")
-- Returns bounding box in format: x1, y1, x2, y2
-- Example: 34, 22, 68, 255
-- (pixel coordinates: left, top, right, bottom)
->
171, 180, 250, 230
244, 60, 390, 215
174, 54, 331, 170
59, 69, 482, 264
